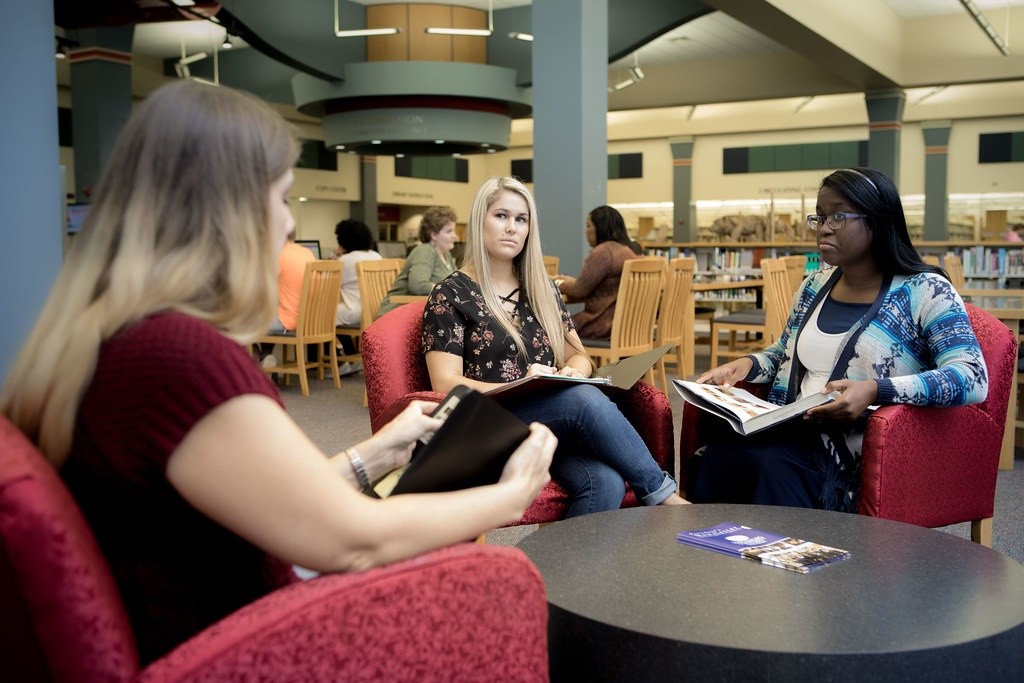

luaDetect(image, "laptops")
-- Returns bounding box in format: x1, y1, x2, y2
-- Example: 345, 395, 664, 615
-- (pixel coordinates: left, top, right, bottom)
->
67, 201, 95, 235
294, 240, 322, 261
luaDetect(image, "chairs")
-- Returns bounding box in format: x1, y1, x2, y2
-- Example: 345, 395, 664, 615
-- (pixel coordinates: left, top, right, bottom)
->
0, 255, 1014, 683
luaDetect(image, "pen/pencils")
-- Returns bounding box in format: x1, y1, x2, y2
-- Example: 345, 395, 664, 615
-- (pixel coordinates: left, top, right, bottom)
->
528, 364, 531, 366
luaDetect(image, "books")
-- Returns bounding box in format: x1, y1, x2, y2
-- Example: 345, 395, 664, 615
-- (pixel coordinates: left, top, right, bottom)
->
645, 248, 827, 303
671, 380, 841, 436
918, 245, 1024, 310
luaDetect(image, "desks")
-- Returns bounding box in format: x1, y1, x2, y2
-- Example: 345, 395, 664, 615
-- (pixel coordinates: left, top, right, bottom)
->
391, 294, 584, 304
517, 505, 1024, 683
661, 278, 764, 340
956, 290, 1024, 470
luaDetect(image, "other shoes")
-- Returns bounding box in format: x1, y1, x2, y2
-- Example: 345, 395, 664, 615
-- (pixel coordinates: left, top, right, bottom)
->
258, 355, 278, 380
350, 360, 364, 371
323, 362, 352, 379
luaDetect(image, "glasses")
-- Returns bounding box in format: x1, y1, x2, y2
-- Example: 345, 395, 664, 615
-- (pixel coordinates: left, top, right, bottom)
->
806, 211, 870, 230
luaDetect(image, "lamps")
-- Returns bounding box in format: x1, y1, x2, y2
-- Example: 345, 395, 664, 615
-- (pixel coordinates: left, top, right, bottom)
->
222, 35, 232, 48
56, 40, 65, 59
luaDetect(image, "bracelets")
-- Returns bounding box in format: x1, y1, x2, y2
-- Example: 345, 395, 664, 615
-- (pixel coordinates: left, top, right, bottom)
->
555, 280, 565, 286
344, 448, 369, 490
328, 251, 338, 261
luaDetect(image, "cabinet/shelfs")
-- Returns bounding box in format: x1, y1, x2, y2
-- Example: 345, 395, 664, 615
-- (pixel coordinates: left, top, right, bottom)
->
641, 242, 1024, 318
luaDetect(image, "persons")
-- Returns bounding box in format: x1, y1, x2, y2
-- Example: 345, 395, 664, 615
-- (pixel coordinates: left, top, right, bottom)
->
684, 167, 989, 511
318, 219, 383, 378
251, 234, 318, 376
375, 208, 459, 324
1006, 223, 1024, 243
0, 78, 559, 672
553, 205, 646, 341
423, 177, 691, 520
